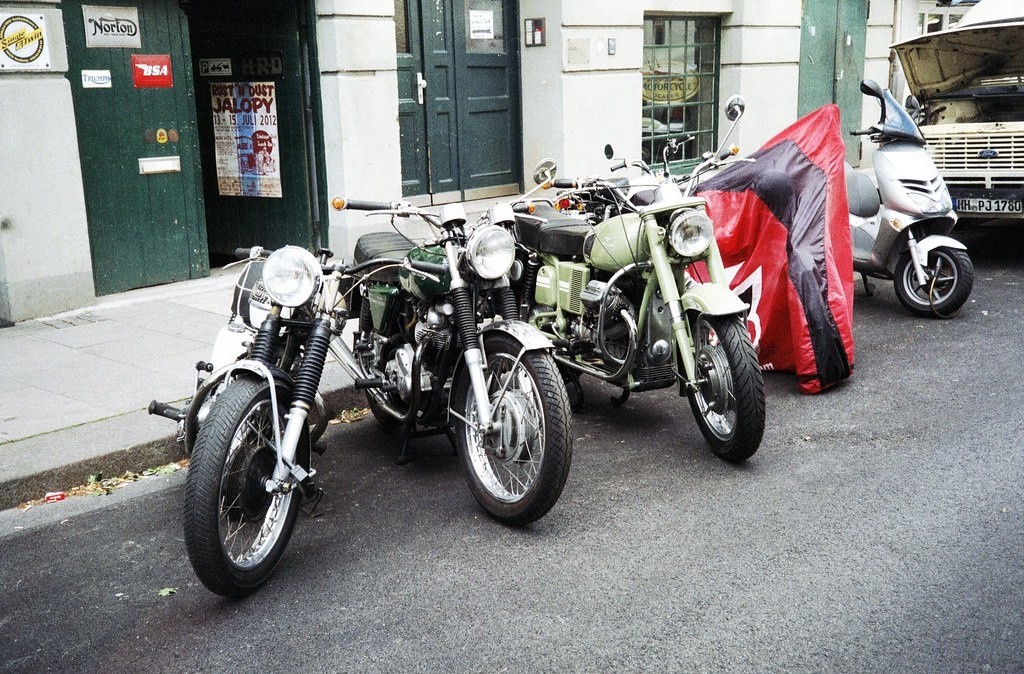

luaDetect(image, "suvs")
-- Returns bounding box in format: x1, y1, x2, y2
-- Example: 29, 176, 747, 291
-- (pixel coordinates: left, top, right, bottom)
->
887, 0, 1023, 240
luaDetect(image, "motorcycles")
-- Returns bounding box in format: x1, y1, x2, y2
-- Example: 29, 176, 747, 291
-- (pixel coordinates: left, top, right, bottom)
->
147, 246, 450, 599
474, 93, 768, 465
327, 156, 581, 527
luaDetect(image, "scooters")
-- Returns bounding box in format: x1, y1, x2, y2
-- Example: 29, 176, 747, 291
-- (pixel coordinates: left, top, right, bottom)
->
734, 77, 974, 319
